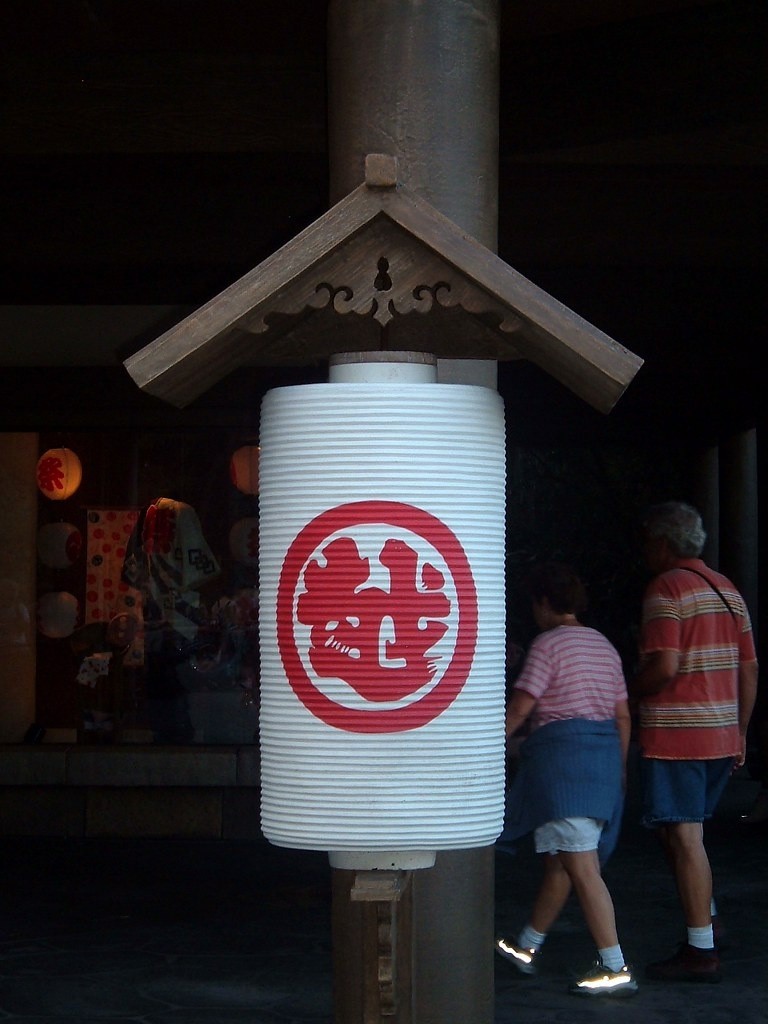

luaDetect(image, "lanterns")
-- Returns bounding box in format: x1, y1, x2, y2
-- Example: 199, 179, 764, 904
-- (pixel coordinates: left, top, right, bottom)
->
229, 446, 258, 496
37, 522, 82, 569
36, 447, 82, 500
38, 591, 80, 638
234, 588, 259, 623
229, 516, 259, 561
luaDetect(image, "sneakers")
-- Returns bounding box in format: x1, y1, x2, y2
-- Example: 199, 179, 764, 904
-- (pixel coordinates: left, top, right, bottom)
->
645, 944, 723, 984
655, 913, 732, 949
495, 934, 544, 976
567, 951, 639, 1000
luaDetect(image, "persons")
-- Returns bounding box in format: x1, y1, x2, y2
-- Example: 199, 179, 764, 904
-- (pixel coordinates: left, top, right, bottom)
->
119, 496, 224, 746
495, 562, 639, 996
627, 502, 759, 982
